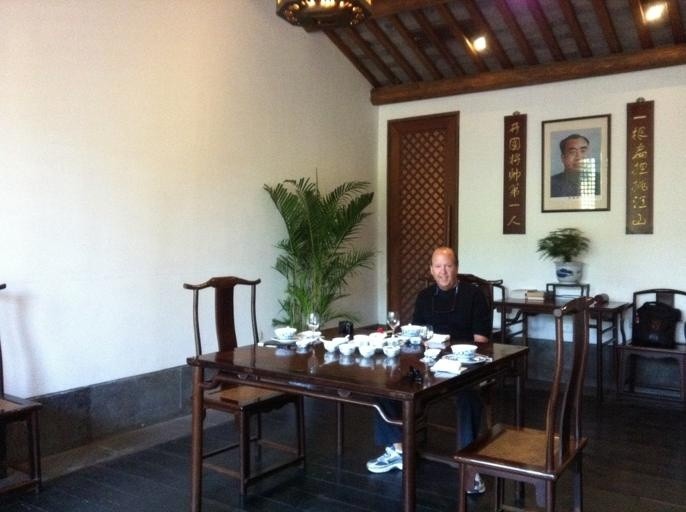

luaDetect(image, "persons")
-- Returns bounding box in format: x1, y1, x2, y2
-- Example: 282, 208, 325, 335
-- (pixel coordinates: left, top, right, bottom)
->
366, 246, 492, 494
548, 133, 601, 196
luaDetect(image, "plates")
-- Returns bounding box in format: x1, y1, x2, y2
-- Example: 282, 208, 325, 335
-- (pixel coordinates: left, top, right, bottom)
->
442, 353, 486, 366
272, 331, 322, 346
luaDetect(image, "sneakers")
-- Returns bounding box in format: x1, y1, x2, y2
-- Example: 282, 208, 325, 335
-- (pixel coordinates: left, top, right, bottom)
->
466, 473, 486, 496
366, 446, 404, 473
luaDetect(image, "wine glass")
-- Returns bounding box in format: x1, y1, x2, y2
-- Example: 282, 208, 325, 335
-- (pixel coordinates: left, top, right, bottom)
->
387, 311, 400, 341
420, 326, 434, 363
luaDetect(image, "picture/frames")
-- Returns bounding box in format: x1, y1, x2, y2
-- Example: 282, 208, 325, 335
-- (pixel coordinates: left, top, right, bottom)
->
541, 114, 611, 212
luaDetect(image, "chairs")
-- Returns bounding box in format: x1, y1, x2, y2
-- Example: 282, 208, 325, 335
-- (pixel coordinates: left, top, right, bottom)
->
182, 277, 304, 497
425, 275, 503, 468
615, 288, 686, 404
0, 284, 43, 495
492, 285, 511, 343
453, 296, 593, 512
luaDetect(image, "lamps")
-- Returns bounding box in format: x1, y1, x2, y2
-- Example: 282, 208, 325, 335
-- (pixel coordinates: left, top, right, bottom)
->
276, 0, 373, 33
638, 0, 668, 23
464, 27, 487, 54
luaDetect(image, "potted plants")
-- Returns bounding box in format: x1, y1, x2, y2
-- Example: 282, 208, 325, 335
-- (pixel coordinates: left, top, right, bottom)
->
536, 227, 590, 284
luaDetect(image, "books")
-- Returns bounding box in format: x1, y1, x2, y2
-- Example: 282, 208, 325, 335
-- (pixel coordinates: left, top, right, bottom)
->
510, 288, 546, 301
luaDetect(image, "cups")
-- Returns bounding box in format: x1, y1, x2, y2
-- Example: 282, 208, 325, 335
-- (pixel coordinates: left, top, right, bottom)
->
306, 312, 320, 331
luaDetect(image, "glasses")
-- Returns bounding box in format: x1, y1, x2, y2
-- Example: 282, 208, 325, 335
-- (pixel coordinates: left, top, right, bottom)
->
431, 291, 457, 315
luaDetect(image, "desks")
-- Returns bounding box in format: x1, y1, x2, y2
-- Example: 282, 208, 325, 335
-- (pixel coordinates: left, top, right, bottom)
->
493, 295, 628, 402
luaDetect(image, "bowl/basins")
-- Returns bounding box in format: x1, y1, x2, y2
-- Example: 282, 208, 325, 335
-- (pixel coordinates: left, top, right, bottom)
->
274, 327, 296, 339
451, 344, 478, 361
321, 333, 402, 359
401, 325, 421, 336
